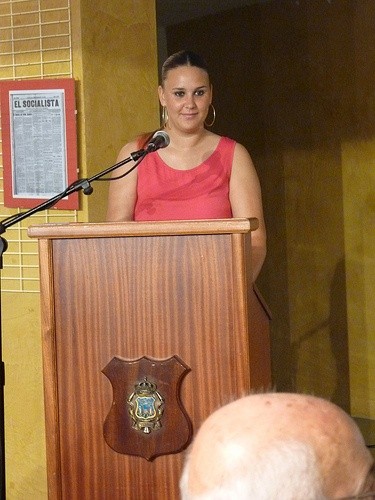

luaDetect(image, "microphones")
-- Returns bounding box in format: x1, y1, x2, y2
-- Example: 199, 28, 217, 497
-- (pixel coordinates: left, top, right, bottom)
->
146, 131, 170, 152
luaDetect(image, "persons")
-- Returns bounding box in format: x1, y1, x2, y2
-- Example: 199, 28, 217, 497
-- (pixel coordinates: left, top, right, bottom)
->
179, 392, 375, 500
106, 51, 267, 287
